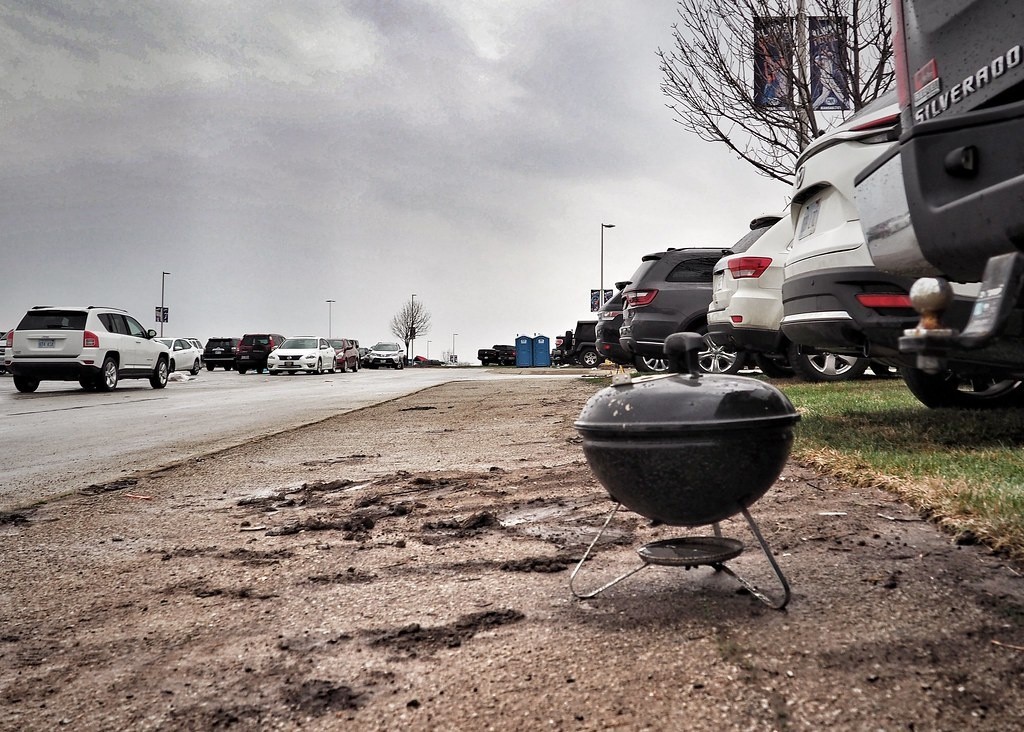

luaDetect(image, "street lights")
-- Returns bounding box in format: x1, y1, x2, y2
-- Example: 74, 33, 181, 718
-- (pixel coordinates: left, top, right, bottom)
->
453, 334, 458, 363
600, 223, 616, 308
326, 300, 336, 339
412, 294, 417, 366
427, 341, 432, 359
161, 271, 170, 337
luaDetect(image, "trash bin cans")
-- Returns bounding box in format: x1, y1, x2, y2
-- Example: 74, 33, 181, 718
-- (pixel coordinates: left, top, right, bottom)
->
514, 335, 533, 368
532, 335, 551, 367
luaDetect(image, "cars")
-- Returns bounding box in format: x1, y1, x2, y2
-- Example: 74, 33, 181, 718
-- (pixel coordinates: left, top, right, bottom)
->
152, 337, 204, 375
267, 337, 337, 375
370, 341, 404, 369
327, 339, 369, 373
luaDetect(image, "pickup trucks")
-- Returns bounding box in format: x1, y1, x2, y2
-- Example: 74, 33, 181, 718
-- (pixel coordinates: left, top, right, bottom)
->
477, 345, 516, 366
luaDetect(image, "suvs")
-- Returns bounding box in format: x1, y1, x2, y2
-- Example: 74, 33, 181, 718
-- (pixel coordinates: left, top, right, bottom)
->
236, 333, 286, 374
203, 337, 242, 371
0, 305, 171, 393
707, 214, 897, 382
780, 1, 1024, 409
553, 248, 732, 373
